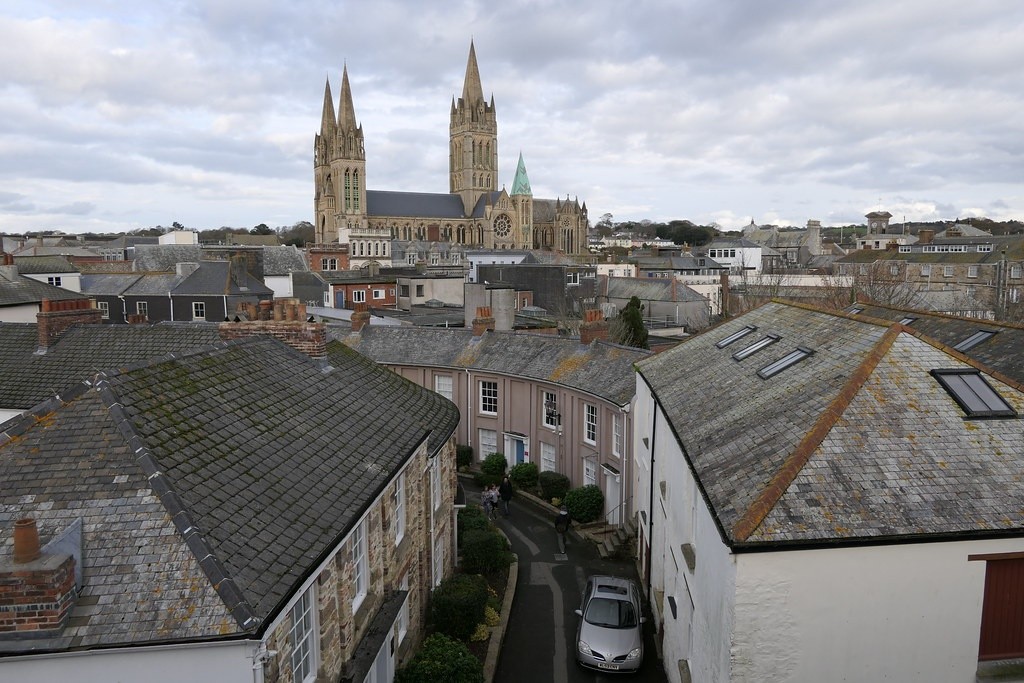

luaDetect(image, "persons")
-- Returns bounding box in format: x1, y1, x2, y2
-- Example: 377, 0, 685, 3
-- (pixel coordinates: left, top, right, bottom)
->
555, 506, 571, 554
481, 477, 513, 521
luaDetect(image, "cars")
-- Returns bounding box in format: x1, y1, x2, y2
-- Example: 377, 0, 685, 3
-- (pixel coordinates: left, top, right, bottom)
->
574, 574, 647, 675
